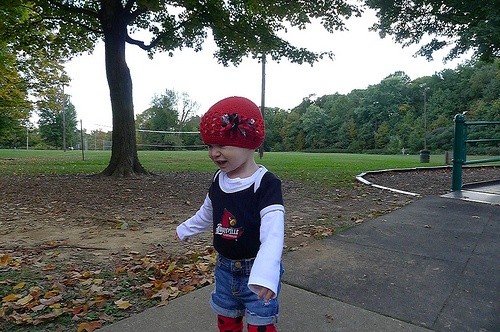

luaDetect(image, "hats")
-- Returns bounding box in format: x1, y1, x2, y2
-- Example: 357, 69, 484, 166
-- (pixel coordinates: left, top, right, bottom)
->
199, 95, 265, 150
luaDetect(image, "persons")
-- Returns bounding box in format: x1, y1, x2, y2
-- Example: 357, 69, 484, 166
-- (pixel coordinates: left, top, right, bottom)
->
174, 96, 286, 332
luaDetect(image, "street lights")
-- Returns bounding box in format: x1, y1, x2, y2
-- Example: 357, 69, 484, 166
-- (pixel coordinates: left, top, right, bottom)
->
57, 83, 69, 152
